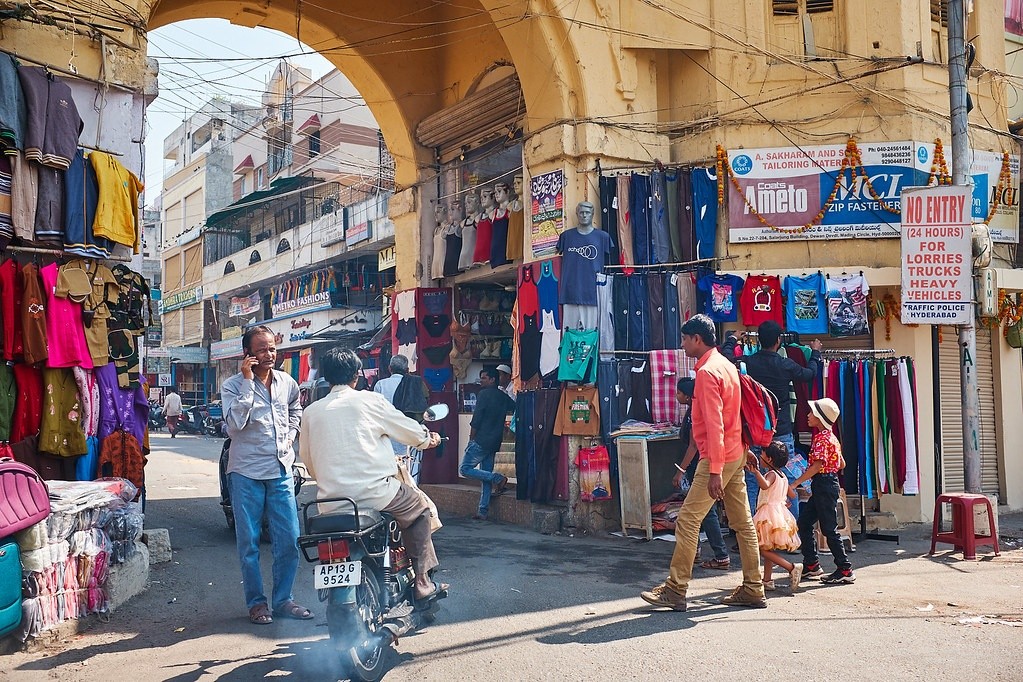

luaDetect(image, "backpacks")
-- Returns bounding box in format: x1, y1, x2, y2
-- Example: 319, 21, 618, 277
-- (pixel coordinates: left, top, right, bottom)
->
737, 368, 778, 448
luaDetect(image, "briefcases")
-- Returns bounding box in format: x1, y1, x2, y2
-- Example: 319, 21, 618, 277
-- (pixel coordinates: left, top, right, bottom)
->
0, 457, 51, 540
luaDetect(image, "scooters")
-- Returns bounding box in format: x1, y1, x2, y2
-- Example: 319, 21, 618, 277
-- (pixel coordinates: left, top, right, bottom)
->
218, 419, 312, 542
146, 396, 227, 438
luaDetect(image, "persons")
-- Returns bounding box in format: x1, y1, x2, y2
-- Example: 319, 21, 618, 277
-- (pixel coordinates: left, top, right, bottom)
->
162, 387, 185, 438
460, 368, 517, 520
745, 441, 804, 592
722, 320, 822, 554
495, 364, 511, 397
430, 173, 524, 281
556, 202, 615, 306
221, 326, 315, 624
790, 398, 856, 585
298, 346, 451, 600
373, 351, 430, 488
640, 312, 767, 612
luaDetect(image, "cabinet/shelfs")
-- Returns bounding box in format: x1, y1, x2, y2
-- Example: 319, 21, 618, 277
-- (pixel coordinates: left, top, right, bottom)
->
615, 436, 697, 539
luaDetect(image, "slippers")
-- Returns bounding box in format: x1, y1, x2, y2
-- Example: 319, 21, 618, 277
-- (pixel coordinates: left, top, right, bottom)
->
415, 581, 446, 605
249, 602, 273, 623
271, 600, 315, 619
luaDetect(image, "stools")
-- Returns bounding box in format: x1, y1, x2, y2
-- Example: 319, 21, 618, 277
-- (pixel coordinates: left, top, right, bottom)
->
794, 487, 856, 553
928, 492, 999, 560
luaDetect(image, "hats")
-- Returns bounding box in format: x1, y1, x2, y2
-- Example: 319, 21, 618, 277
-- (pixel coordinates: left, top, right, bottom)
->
808, 397, 840, 430
495, 365, 511, 374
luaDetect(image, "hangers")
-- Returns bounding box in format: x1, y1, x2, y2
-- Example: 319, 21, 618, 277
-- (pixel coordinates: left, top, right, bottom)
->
79, 143, 111, 159
11, 47, 49, 71
452, 283, 517, 347
573, 156, 895, 454
12, 245, 129, 272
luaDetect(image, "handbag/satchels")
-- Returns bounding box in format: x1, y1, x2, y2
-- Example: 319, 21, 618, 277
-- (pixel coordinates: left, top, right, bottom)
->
107, 328, 135, 359
62, 267, 92, 303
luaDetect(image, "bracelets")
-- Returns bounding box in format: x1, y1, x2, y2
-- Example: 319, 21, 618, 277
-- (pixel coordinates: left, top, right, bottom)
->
674, 463, 686, 473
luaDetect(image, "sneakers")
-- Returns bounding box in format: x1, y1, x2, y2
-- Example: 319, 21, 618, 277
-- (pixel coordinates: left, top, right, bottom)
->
762, 579, 775, 591
801, 562, 824, 577
722, 585, 767, 608
640, 584, 686, 612
820, 567, 856, 583
789, 562, 803, 592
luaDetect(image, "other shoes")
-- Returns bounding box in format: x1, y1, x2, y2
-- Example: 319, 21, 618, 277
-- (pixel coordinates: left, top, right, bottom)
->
495, 477, 508, 494
731, 543, 740, 553
469, 512, 488, 521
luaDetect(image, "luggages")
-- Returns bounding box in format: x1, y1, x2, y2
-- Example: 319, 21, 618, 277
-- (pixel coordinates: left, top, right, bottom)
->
1, 541, 24, 638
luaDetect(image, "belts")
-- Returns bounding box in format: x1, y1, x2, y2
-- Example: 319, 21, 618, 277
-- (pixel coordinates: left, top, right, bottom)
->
811, 472, 834, 478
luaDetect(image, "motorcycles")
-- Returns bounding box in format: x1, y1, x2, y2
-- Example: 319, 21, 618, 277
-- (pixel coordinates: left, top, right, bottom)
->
294, 400, 452, 682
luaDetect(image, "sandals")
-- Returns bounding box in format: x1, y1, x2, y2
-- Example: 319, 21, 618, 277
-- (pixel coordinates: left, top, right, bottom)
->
701, 559, 730, 570
693, 554, 702, 563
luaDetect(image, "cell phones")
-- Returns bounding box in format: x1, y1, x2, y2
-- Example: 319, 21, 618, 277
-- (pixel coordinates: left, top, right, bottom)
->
244, 353, 257, 370
